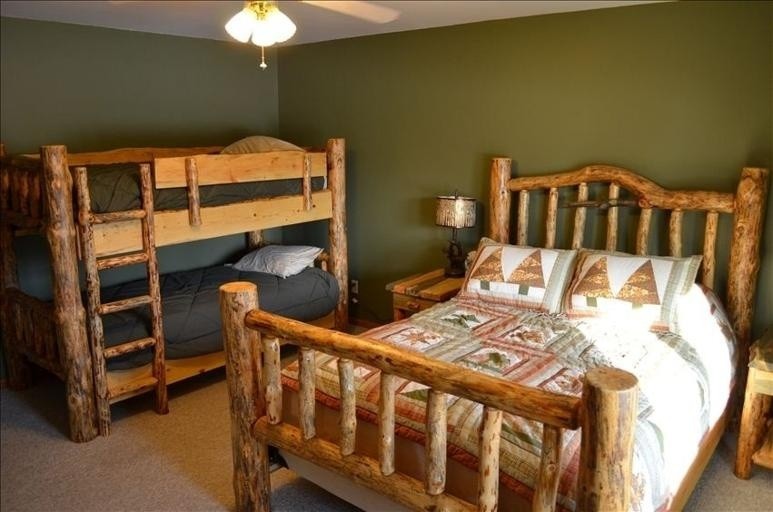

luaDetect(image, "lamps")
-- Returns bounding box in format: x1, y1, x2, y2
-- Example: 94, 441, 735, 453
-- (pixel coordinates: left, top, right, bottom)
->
224, 0, 297, 71
434, 189, 477, 277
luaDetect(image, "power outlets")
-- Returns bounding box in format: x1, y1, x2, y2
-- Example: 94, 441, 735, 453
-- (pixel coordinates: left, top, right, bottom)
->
351, 281, 359, 294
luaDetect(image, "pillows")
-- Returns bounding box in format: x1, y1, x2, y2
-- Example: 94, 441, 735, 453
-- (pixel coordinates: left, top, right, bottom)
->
232, 245, 324, 279
221, 135, 306, 154
561, 247, 702, 335
460, 236, 576, 315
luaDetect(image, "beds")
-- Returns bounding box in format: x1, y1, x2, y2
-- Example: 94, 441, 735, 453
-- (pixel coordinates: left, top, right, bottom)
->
0, 138, 348, 444
218, 157, 769, 512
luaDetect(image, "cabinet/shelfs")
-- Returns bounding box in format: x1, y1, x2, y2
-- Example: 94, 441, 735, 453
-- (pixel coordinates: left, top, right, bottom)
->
385, 266, 464, 321
732, 330, 773, 479
